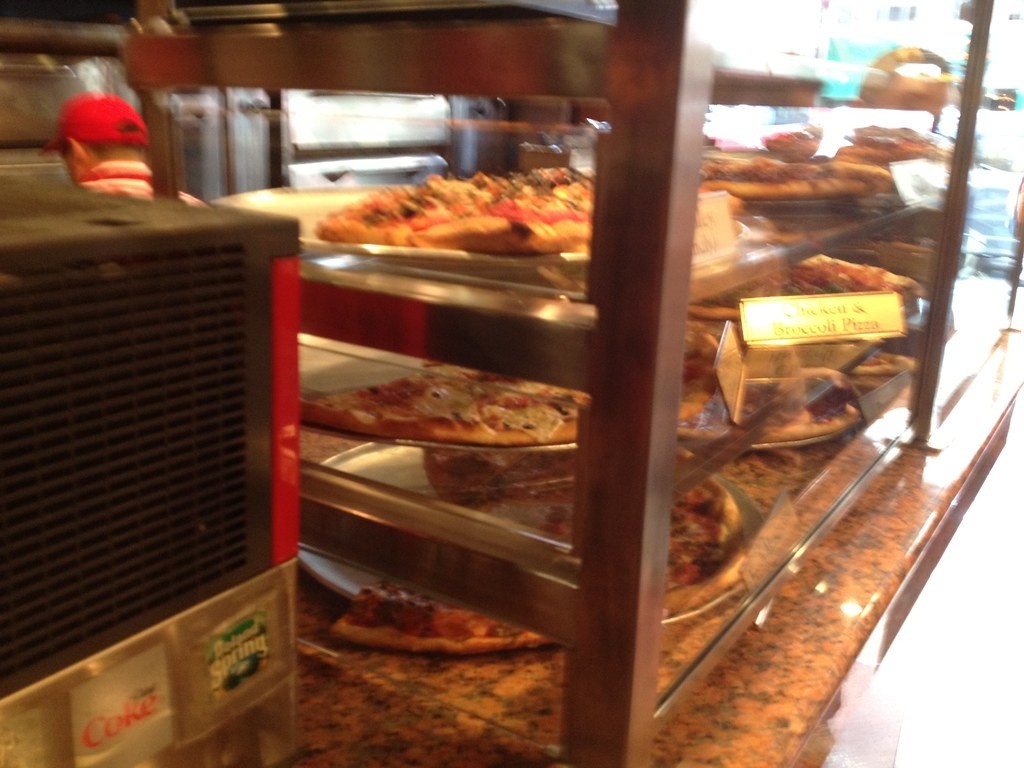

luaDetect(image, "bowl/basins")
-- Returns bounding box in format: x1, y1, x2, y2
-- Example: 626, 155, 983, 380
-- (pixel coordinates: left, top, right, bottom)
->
759, 126, 824, 158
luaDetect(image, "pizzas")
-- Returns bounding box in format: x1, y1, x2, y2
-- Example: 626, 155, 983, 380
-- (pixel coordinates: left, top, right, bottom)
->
297, 128, 957, 657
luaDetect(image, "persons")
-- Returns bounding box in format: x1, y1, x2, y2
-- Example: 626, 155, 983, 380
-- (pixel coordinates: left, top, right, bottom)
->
37, 94, 213, 213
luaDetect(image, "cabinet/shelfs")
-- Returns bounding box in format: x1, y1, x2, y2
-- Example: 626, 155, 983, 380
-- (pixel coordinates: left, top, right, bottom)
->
122, 0, 992, 768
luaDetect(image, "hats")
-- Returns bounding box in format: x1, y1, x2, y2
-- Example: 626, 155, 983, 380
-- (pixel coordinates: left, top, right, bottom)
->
39, 91, 151, 156
